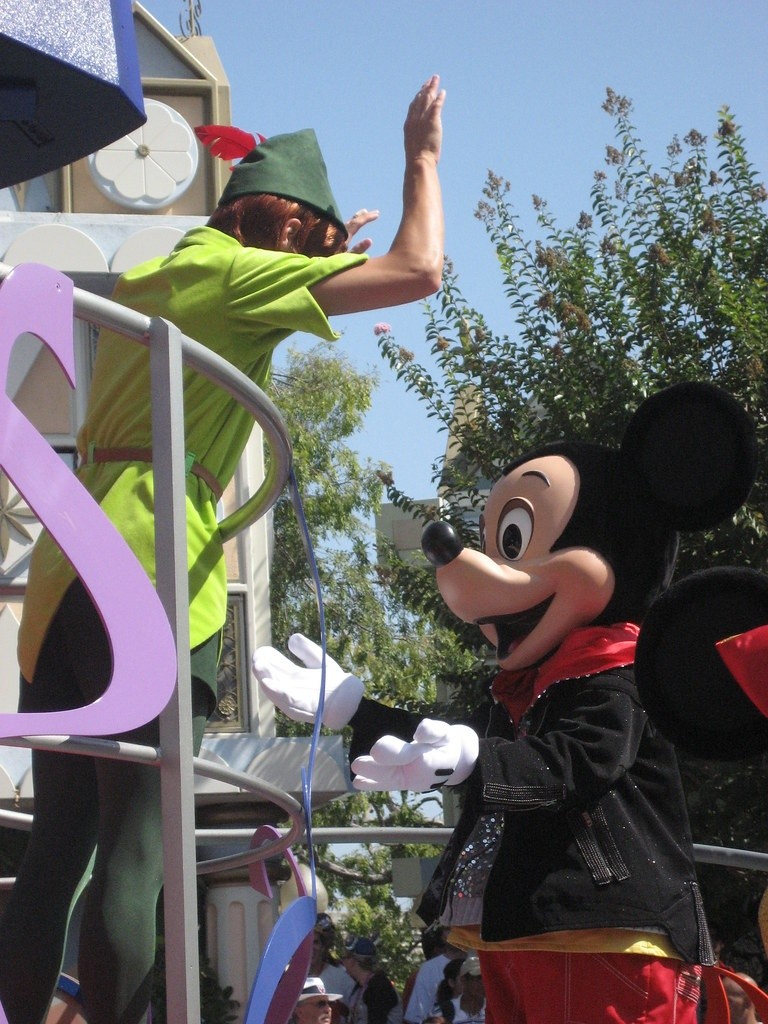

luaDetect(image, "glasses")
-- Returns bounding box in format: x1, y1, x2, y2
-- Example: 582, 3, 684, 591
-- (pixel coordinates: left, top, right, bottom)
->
300, 1000, 334, 1009
465, 974, 483, 981
314, 940, 323, 946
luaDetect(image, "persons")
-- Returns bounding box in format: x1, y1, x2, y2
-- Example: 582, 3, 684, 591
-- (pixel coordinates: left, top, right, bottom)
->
401, 925, 487, 1024
285, 977, 343, 1024
308, 913, 404, 1024
17, 72, 449, 1024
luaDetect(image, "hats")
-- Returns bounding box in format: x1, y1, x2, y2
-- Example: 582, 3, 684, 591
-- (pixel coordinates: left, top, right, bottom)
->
315, 914, 335, 949
296, 977, 343, 1005
349, 937, 378, 963
460, 957, 483, 978
192, 123, 348, 241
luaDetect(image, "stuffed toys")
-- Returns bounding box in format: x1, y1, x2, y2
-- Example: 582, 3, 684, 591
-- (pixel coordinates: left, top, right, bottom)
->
249, 382, 768, 1024
